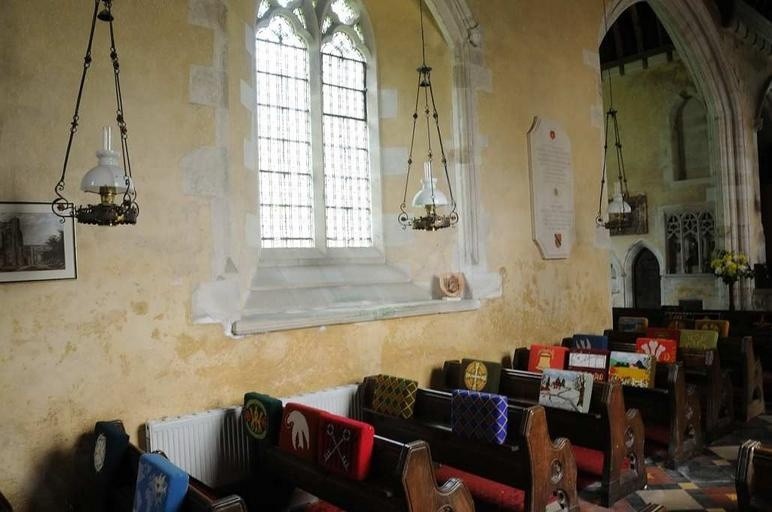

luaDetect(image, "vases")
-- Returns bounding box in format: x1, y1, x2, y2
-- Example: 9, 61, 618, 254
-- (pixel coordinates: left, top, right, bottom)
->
729, 283, 735, 308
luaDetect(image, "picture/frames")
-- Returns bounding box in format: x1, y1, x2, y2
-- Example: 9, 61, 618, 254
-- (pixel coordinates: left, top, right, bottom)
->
0, 202, 77, 283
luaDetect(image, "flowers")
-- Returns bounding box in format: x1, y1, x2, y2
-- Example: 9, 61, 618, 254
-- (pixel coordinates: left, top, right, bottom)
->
711, 250, 754, 283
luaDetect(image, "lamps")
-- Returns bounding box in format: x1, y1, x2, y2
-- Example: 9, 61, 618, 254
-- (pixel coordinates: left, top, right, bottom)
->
596, 1, 631, 230
398, 0, 459, 231
51, 0, 141, 224
